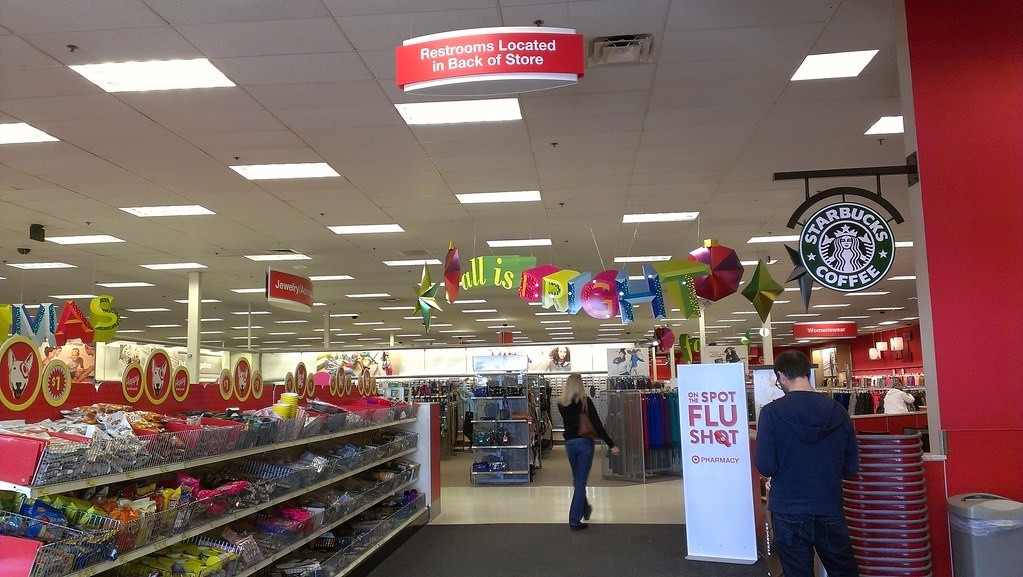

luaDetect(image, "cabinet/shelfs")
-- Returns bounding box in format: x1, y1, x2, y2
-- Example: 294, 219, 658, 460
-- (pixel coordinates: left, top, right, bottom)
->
0, 375, 554, 577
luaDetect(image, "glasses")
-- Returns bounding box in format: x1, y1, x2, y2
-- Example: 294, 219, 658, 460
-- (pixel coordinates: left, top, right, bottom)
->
775, 377, 782, 390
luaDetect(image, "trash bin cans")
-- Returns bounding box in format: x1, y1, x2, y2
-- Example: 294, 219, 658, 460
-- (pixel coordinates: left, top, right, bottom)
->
947, 491, 1023, 577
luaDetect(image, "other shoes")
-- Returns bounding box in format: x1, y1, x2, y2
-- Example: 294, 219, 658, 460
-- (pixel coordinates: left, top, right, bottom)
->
570, 522, 588, 529
584, 504, 591, 519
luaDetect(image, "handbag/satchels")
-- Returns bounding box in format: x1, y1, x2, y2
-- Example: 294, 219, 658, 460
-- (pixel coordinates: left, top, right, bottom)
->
489, 461, 508, 472
474, 385, 522, 397
479, 417, 496, 420
500, 409, 509, 420
473, 429, 493, 446
472, 462, 490, 472
577, 396, 601, 441
511, 412, 533, 420
491, 427, 511, 445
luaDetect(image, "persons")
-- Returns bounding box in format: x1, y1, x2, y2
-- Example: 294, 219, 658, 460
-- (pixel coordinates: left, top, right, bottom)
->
612, 348, 645, 375
555, 373, 622, 530
753, 349, 864, 577
379, 351, 393, 375
455, 380, 476, 453
725, 347, 741, 363
545, 346, 572, 371
881, 382, 916, 415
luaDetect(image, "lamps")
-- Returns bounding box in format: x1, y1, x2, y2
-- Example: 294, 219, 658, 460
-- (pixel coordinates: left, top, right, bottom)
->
869, 331, 881, 359
876, 331, 887, 351
890, 328, 903, 351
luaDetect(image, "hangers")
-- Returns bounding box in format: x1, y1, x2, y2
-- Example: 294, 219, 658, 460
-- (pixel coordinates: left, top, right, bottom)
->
834, 388, 887, 393
